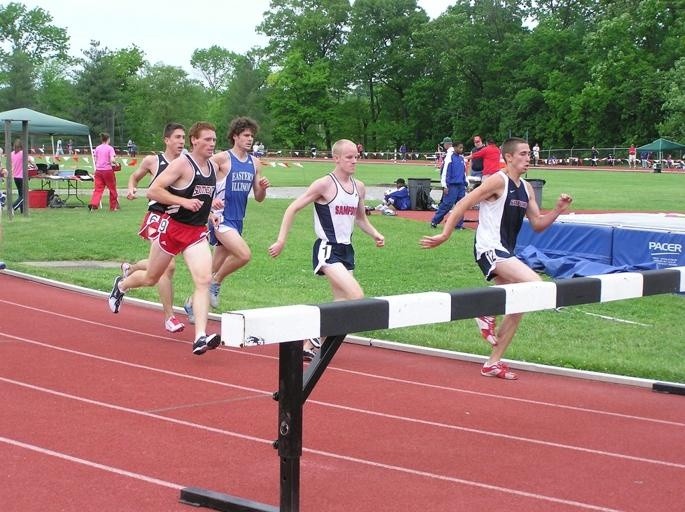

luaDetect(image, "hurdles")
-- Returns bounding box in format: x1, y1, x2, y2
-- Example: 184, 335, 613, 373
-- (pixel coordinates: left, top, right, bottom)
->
179, 265, 685, 512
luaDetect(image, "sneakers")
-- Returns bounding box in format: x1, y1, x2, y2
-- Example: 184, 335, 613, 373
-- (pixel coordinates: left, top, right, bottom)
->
302, 350, 315, 360
208, 272, 221, 308
473, 315, 499, 347
120, 263, 131, 291
183, 298, 195, 324
192, 334, 220, 356
431, 222, 437, 227
480, 361, 518, 380
310, 337, 321, 348
164, 316, 184, 333
455, 226, 466, 231
374, 204, 388, 211
109, 276, 125, 313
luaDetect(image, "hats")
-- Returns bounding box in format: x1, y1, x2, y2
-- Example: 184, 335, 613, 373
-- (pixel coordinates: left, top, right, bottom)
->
394, 178, 405, 184
440, 137, 452, 145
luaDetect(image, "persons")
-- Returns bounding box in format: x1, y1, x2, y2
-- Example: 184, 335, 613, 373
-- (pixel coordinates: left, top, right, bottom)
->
429, 136, 501, 230
0, 132, 265, 215
533, 143, 540, 166
419, 137, 572, 379
268, 139, 385, 362
591, 143, 685, 169
108, 121, 220, 355
183, 117, 270, 324
121, 123, 187, 333
400, 143, 407, 162
375, 178, 410, 211
310, 143, 316, 159
358, 143, 363, 159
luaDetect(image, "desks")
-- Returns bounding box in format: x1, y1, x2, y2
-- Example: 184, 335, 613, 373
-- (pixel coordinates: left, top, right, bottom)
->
27, 175, 94, 208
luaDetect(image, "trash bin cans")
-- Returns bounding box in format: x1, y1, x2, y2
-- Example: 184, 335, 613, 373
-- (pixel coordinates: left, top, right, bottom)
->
524, 179, 546, 209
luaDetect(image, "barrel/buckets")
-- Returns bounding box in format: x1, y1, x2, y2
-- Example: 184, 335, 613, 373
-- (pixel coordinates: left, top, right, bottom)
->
523, 177, 546, 210
